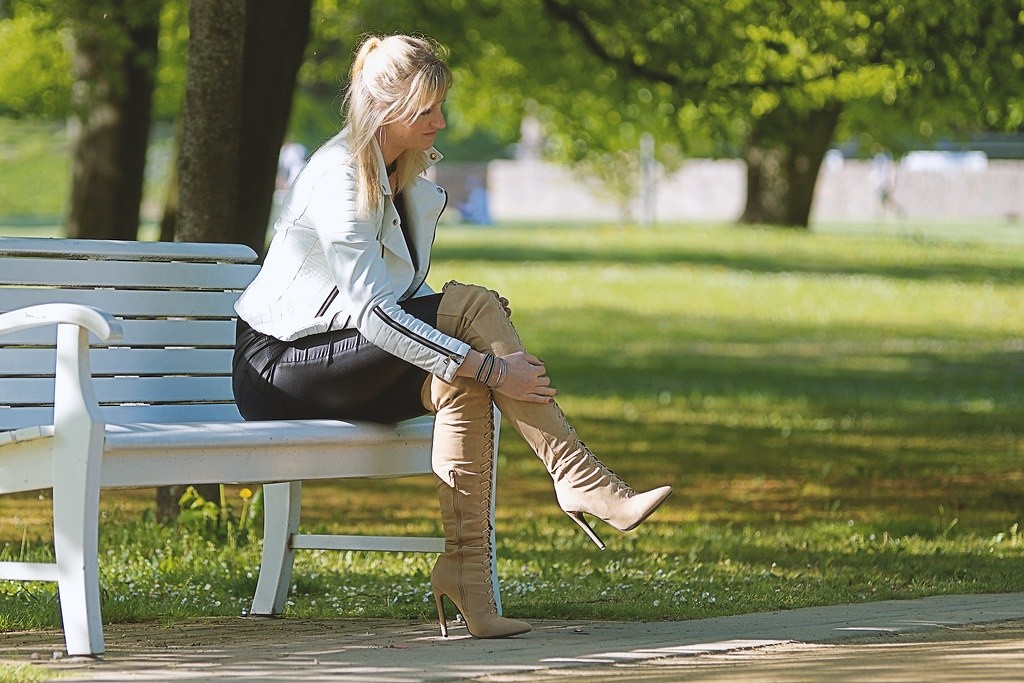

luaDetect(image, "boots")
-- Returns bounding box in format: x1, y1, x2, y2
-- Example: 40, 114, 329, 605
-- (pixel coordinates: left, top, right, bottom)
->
418, 277, 671, 641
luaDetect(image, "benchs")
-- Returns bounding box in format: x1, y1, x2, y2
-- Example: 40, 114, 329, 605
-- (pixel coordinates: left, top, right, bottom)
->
0, 235, 514, 662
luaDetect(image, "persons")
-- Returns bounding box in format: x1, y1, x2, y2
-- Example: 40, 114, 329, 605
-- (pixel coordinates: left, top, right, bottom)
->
233, 32, 675, 637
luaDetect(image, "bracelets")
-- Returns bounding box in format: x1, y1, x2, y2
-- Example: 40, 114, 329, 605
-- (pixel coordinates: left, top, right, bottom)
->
475, 353, 507, 391
